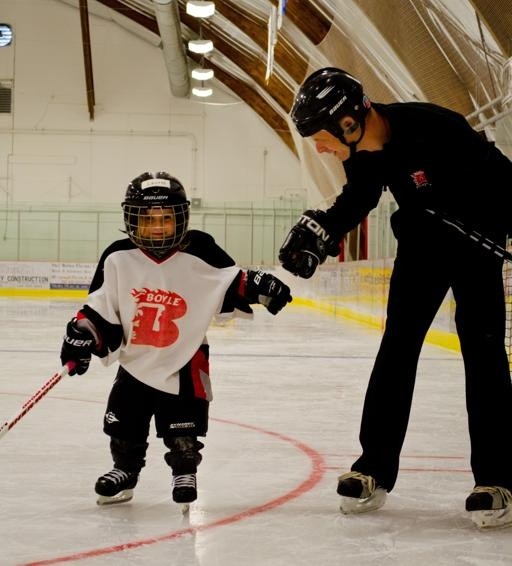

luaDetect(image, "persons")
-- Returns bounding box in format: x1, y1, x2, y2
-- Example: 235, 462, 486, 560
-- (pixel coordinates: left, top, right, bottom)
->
277, 66, 512, 512
60, 172, 292, 503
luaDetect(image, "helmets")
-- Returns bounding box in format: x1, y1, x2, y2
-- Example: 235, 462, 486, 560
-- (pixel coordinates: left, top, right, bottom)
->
291, 67, 371, 137
119, 172, 190, 252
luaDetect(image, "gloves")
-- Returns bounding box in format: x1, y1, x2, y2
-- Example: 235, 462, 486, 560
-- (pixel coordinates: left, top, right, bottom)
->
279, 210, 342, 279
61, 317, 93, 376
245, 270, 292, 315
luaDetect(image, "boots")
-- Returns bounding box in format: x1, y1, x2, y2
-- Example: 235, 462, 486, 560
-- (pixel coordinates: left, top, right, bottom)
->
172, 474, 197, 503
465, 486, 512, 512
95, 468, 138, 496
337, 471, 380, 498
